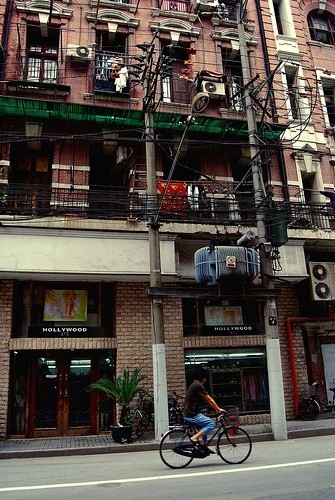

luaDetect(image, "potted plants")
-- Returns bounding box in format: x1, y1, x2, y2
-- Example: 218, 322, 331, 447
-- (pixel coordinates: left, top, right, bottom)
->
66, 43, 92, 64
86, 365, 151, 445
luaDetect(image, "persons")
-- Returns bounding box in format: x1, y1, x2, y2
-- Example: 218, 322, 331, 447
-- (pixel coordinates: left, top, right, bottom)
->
182, 371, 226, 454
13, 375, 26, 434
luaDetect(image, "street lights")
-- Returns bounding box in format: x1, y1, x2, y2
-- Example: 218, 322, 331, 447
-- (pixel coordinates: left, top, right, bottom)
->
148, 91, 209, 443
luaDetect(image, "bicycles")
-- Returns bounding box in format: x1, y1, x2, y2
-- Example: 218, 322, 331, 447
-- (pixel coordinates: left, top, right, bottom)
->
159, 404, 252, 469
131, 391, 185, 438
297, 378, 335, 421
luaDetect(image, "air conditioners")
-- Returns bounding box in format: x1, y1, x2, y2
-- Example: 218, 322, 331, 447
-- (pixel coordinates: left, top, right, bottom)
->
130, 190, 148, 210
200, 81, 226, 100
211, 199, 242, 220
308, 260, 335, 303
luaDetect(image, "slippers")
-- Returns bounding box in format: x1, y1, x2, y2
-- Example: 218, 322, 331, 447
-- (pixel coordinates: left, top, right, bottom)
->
202, 446, 214, 453
190, 439, 201, 447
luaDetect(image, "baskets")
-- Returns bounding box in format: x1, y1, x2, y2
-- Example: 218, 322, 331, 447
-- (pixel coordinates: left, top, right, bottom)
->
223, 409, 240, 426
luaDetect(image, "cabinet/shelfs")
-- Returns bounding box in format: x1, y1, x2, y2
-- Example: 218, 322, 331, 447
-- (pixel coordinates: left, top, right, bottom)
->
241, 366, 270, 414
209, 366, 244, 414
